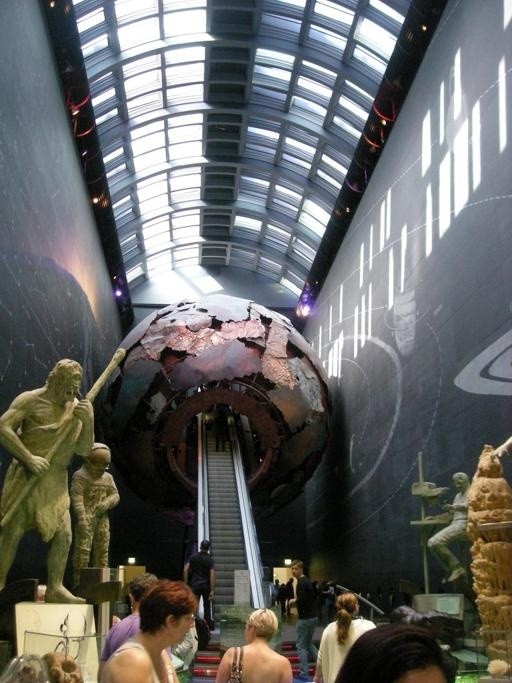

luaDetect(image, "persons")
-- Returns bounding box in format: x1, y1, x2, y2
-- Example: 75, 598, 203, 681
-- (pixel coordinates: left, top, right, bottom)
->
288, 560, 376, 682
184, 540, 215, 630
217, 608, 293, 682
200, 378, 231, 451
313, 593, 455, 683
69, 442, 120, 591
97, 574, 198, 683
273, 578, 296, 616
0, 357, 96, 604
427, 472, 471, 584
